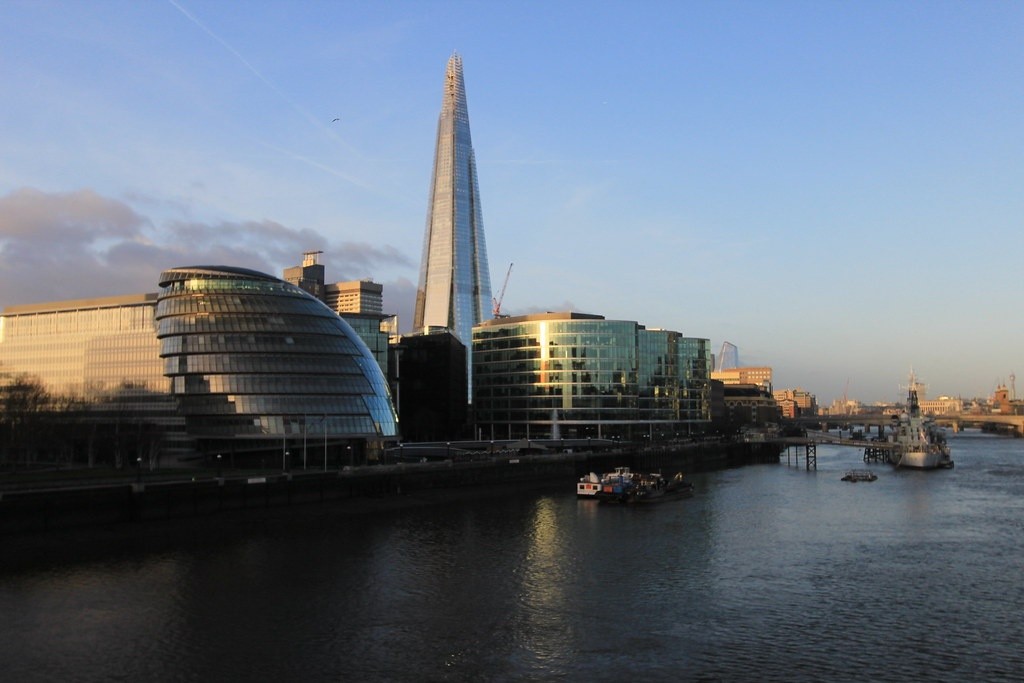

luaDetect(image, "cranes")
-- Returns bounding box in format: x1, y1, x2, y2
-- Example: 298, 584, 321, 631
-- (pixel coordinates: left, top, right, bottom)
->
493, 262, 514, 318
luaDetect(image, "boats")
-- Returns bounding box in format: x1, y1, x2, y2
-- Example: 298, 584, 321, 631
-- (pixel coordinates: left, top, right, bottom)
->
840, 468, 878, 483
888, 389, 955, 470
576, 465, 696, 504
